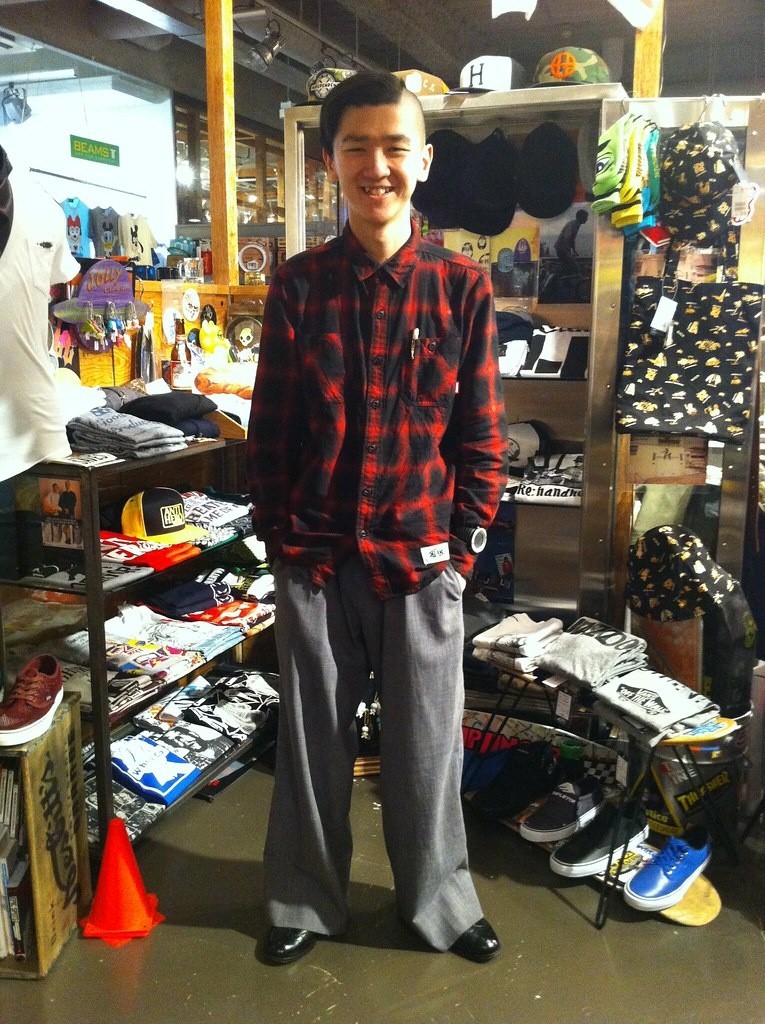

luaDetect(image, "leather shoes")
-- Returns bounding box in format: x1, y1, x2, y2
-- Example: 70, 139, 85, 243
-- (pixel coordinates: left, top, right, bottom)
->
255, 926, 320, 966
450, 916, 501, 960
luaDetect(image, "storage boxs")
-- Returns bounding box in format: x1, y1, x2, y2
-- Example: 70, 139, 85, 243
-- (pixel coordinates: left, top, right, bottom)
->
631, 611, 706, 696
0, 691, 95, 981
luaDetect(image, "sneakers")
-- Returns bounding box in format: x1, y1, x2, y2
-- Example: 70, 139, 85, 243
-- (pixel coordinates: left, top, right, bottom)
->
551, 803, 651, 878
519, 776, 606, 843
622, 823, 715, 912
0, 653, 66, 747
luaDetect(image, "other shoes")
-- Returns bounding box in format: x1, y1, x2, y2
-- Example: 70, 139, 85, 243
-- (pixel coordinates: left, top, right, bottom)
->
476, 745, 545, 817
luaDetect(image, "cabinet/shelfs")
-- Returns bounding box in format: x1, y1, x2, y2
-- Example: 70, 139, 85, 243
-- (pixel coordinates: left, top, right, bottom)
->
0, 438, 277, 861
58, 272, 230, 387
284, 82, 627, 722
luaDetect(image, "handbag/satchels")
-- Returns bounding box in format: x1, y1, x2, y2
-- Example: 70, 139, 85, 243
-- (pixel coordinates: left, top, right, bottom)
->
614, 233, 765, 445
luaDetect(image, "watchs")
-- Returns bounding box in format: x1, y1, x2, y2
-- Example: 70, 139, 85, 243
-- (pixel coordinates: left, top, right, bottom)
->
455, 526, 488, 554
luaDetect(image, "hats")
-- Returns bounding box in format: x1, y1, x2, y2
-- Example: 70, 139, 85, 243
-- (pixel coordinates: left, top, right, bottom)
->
526, 43, 612, 85
291, 68, 361, 109
447, 57, 533, 92
627, 523, 740, 621
660, 120, 743, 247
120, 486, 206, 546
413, 122, 578, 235
492, 422, 546, 476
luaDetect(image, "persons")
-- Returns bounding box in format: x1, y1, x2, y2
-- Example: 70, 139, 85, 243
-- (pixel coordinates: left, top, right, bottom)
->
45, 480, 77, 520
554, 209, 588, 273
244, 69, 511, 965
502, 556, 512, 574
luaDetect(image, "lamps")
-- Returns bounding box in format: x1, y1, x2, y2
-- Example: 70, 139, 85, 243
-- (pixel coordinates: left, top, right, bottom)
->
0, 90, 11, 126
243, 29, 287, 75
3, 96, 32, 125
